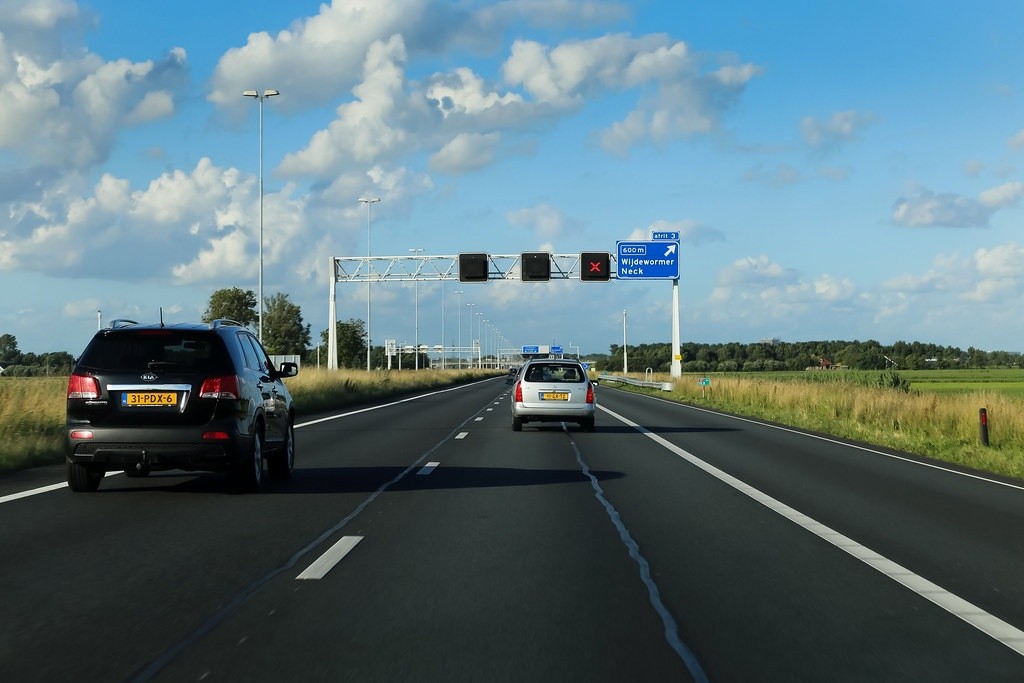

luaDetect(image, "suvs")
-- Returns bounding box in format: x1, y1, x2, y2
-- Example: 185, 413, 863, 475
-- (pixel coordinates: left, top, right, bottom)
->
509, 356, 600, 431
63, 318, 300, 493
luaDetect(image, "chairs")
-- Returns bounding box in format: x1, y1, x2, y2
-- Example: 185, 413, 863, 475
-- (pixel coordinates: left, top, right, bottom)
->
564, 372, 575, 381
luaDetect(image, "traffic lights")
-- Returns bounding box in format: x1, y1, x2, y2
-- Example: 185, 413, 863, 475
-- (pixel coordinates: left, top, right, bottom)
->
521, 252, 550, 282
580, 251, 610, 282
457, 253, 489, 283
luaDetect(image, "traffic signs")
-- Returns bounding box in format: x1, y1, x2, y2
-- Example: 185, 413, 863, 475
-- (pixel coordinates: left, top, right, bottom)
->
615, 239, 680, 280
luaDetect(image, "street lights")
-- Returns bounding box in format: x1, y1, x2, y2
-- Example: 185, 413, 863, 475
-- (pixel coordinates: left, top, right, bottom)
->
622, 307, 628, 374
453, 290, 464, 369
243, 88, 280, 347
407, 248, 426, 370
466, 303, 512, 369
358, 197, 381, 372
